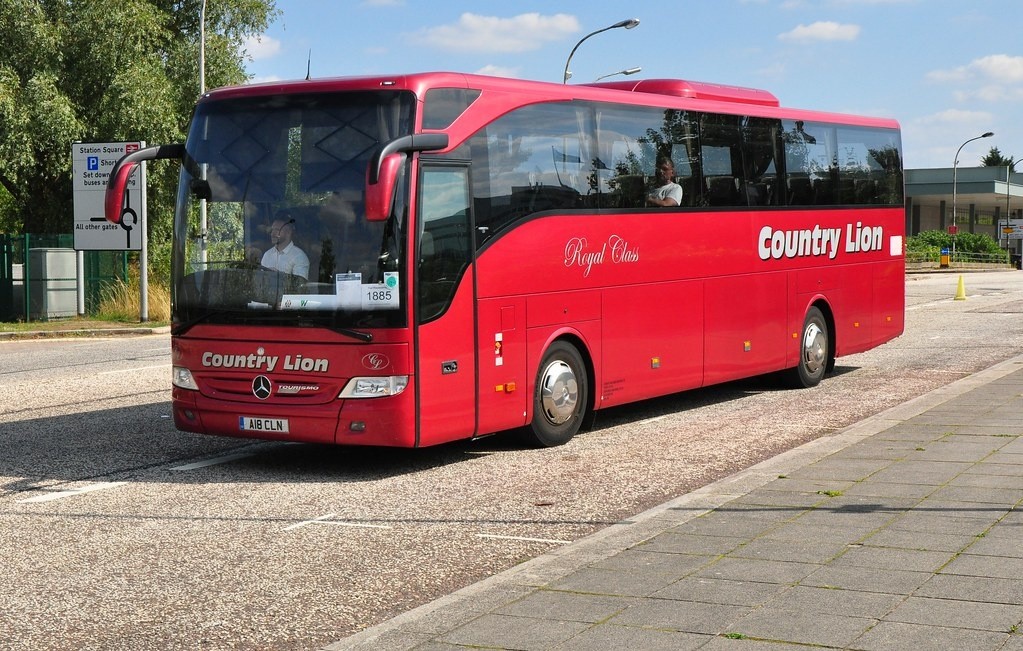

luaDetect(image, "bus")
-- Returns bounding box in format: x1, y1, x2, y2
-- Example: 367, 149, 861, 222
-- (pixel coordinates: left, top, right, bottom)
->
105, 71, 907, 450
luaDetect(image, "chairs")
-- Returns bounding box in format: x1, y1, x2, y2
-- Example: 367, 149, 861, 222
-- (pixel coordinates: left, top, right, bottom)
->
854, 178, 875, 204
496, 171, 536, 217
786, 177, 813, 205
839, 179, 855, 205
708, 176, 741, 206
615, 175, 647, 209
746, 180, 768, 206
543, 172, 577, 211
812, 179, 837, 206
679, 176, 694, 206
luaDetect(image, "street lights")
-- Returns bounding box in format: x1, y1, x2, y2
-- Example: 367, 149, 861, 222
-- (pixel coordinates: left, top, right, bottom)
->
563, 17, 641, 187
952, 131, 995, 263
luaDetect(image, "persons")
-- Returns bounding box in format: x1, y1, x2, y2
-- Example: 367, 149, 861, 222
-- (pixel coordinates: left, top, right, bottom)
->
645, 157, 683, 206
261, 221, 310, 285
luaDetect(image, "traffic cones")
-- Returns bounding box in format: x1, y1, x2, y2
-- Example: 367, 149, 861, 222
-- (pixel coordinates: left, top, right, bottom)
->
954, 274, 966, 300
